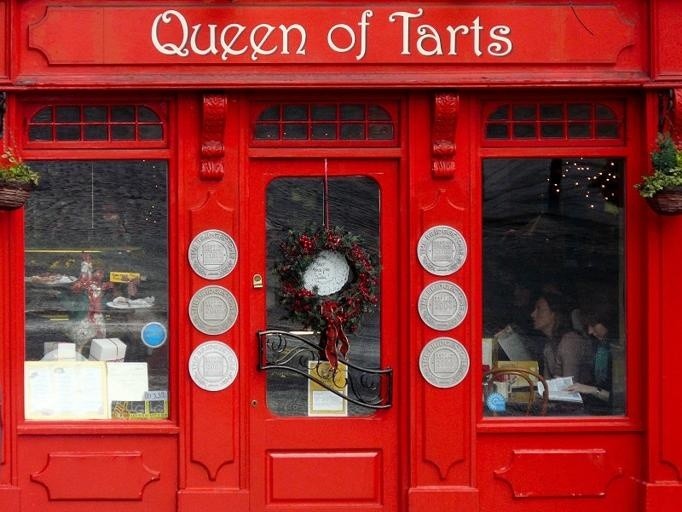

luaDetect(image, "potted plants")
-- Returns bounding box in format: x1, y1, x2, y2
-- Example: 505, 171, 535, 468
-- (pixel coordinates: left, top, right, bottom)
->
0, 147, 40, 210
633, 129, 681, 214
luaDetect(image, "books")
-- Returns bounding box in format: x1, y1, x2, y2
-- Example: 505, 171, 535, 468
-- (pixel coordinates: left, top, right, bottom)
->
536, 375, 587, 404
494, 321, 540, 362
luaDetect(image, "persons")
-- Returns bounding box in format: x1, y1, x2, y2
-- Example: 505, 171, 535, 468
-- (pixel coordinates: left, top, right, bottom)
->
573, 295, 630, 400
530, 291, 596, 388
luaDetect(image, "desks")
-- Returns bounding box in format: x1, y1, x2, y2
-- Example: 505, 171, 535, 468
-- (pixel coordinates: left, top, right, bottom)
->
488, 372, 582, 410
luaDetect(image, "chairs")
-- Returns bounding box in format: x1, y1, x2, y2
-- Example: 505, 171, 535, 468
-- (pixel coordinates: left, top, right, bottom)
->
483, 370, 550, 417
